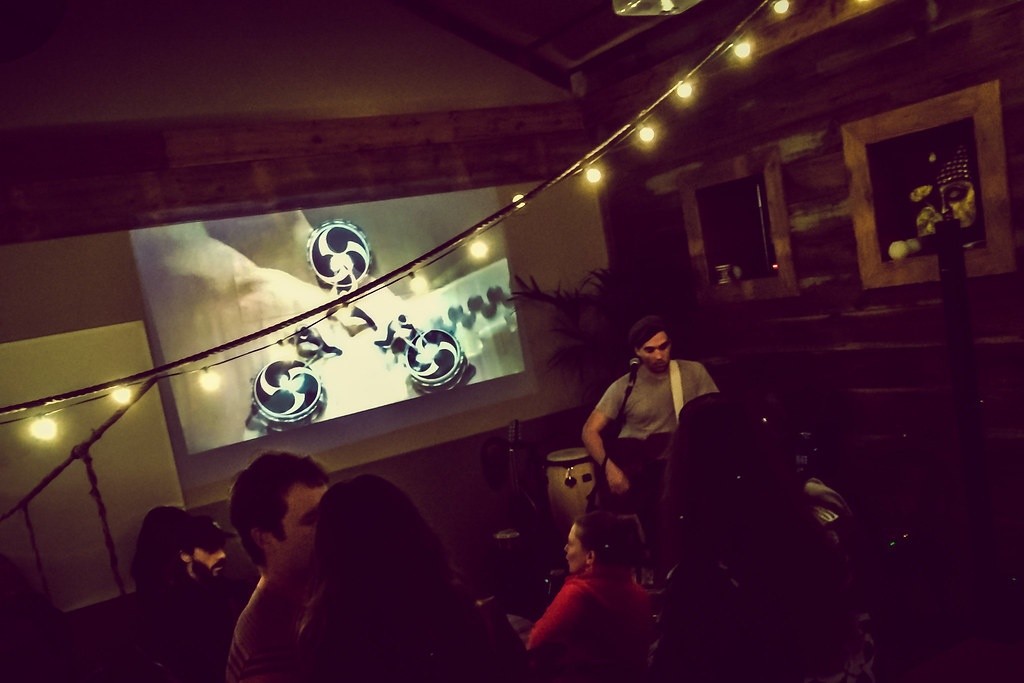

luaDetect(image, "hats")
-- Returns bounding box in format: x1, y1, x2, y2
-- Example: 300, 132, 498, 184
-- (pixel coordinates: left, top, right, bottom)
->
629, 315, 665, 349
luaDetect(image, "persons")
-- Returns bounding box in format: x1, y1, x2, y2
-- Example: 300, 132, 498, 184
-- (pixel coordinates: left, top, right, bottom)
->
936, 143, 983, 248
130, 391, 873, 683
580, 315, 721, 513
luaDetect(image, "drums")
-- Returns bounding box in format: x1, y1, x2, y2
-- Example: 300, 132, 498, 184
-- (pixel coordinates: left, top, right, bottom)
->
545, 446, 600, 524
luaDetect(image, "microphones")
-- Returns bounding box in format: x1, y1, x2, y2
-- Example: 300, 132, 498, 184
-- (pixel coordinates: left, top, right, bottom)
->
626, 357, 640, 395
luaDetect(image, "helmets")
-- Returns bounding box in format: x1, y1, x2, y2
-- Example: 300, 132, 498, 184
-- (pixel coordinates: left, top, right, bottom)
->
180, 515, 236, 551
